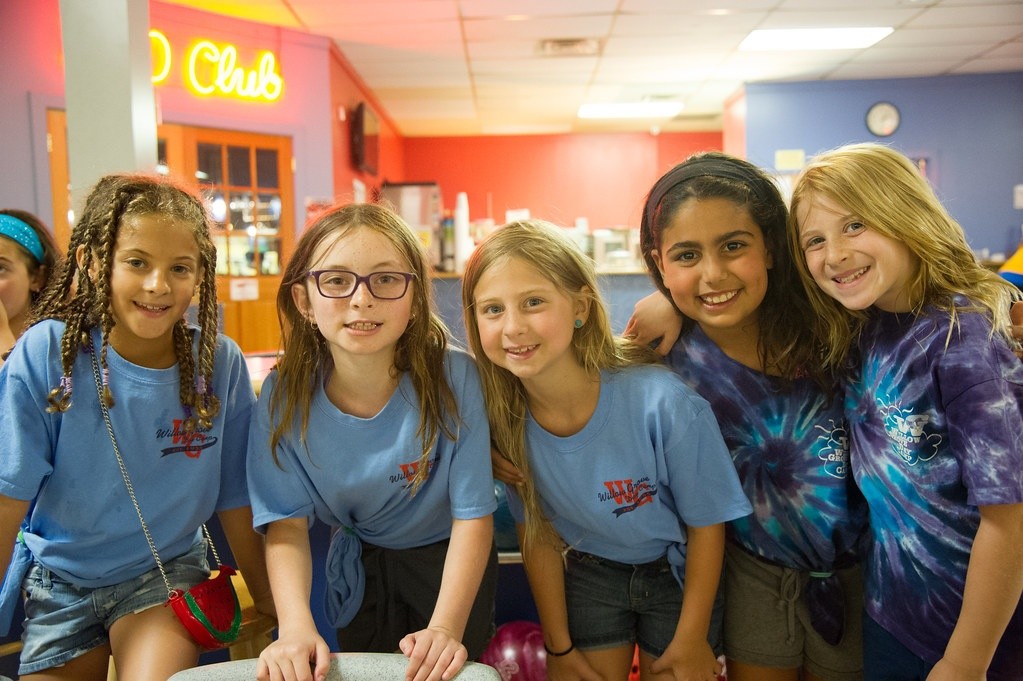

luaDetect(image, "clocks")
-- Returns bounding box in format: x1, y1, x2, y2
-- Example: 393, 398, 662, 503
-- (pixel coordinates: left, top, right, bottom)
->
865, 102, 900, 137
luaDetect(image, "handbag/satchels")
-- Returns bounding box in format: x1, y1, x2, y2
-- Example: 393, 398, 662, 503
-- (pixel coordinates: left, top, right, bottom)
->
164, 565, 244, 651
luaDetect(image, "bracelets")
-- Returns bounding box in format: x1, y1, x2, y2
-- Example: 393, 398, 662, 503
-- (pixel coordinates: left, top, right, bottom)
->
544, 638, 575, 656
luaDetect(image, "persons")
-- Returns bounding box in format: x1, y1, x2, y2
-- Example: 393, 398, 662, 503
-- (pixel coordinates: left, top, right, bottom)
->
620, 143, 1023, 680
1, 174, 277, 680
460, 217, 756, 680
1, 208, 64, 372
638, 150, 870, 680
244, 203, 500, 681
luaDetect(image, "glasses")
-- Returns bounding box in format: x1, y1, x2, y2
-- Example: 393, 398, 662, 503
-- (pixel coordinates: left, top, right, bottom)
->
296, 267, 416, 302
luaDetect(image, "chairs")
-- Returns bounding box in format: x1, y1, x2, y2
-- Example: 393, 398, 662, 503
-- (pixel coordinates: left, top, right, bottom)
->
165, 648, 502, 680
